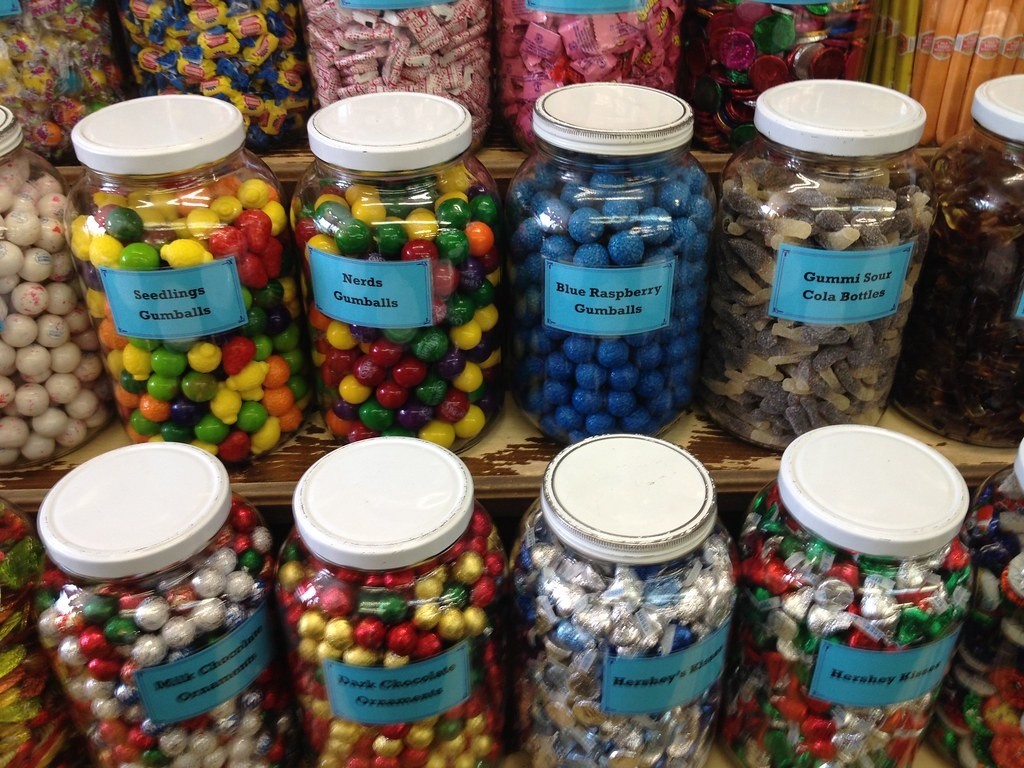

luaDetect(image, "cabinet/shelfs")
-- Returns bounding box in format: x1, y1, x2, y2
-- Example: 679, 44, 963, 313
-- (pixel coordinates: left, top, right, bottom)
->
0, 141, 1024, 768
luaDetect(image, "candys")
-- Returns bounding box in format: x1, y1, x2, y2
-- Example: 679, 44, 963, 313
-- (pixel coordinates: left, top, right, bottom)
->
0, 0, 1024, 768
